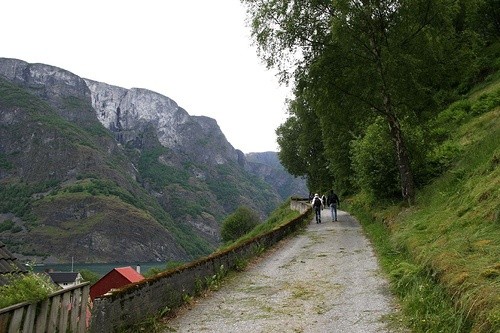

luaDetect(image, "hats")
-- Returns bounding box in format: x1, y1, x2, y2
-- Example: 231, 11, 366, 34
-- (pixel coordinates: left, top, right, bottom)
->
315, 193, 319, 197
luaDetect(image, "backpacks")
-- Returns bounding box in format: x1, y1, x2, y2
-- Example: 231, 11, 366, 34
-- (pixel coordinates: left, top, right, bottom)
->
315, 198, 321, 207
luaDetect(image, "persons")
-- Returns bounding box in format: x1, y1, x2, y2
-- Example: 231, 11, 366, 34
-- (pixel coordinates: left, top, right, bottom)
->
308, 193, 314, 203
320, 194, 326, 209
311, 193, 324, 223
327, 190, 340, 222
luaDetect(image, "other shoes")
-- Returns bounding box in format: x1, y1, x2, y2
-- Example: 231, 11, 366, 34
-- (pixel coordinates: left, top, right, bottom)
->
335, 218, 337, 221
332, 220, 334, 222
316, 220, 318, 224
319, 219, 321, 223
